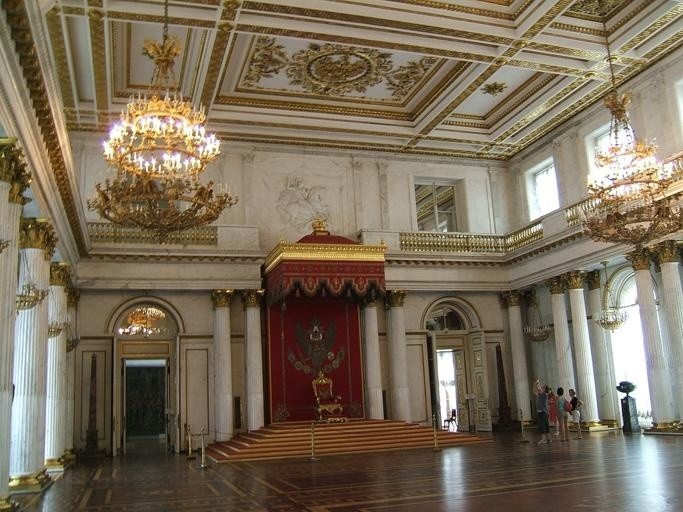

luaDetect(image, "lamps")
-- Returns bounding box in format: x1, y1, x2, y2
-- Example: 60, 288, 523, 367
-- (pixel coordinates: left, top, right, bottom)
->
86, 0, 241, 233
576, 0, 683, 333
118, 307, 168, 338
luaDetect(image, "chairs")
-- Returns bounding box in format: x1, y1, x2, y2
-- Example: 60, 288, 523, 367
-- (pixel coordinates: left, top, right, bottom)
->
443, 408, 459, 433
310, 369, 348, 424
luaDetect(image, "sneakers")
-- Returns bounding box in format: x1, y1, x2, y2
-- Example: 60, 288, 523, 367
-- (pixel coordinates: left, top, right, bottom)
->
575, 436, 582, 439
553, 431, 559, 435
540, 439, 552, 444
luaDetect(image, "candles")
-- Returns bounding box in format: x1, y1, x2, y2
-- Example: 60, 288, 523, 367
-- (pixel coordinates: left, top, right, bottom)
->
523, 325, 551, 332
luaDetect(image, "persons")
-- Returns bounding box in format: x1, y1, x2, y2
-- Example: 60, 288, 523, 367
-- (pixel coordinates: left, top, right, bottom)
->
556, 386, 574, 444
547, 384, 559, 438
567, 387, 584, 441
533, 377, 553, 445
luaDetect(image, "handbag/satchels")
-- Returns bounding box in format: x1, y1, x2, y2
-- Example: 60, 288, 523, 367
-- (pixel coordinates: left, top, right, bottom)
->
565, 401, 572, 412
